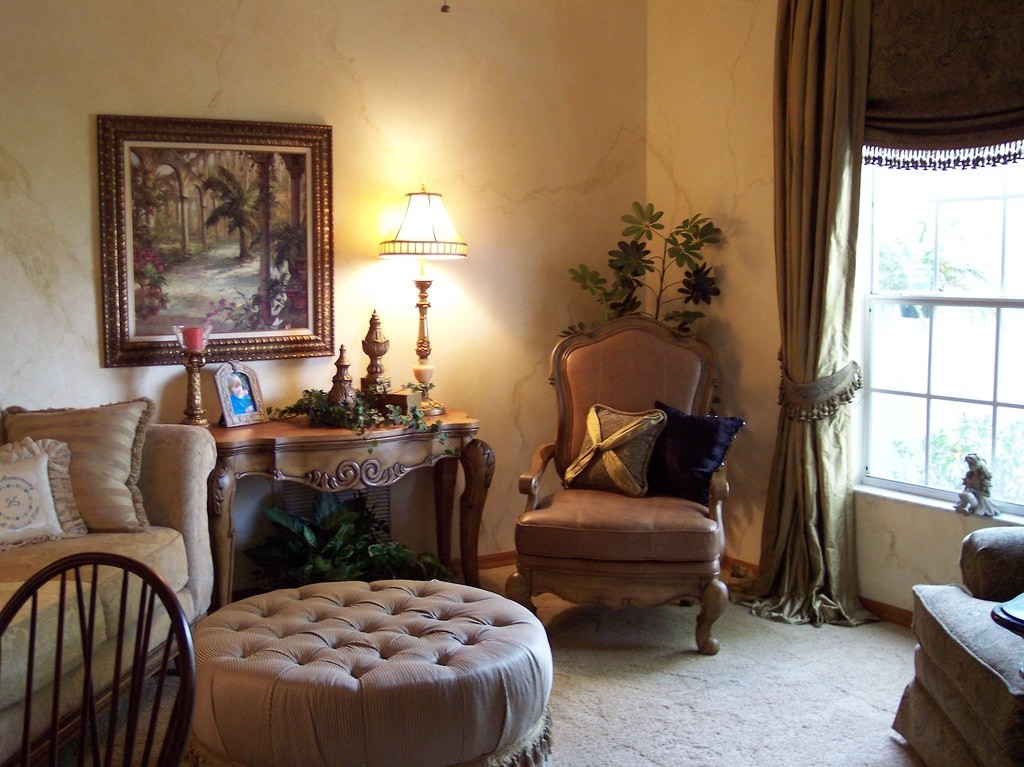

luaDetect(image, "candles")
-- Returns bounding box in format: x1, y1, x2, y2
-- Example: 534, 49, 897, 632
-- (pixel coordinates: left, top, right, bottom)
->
182, 326, 204, 352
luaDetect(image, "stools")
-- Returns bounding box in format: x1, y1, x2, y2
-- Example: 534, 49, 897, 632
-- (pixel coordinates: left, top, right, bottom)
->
184, 580, 554, 767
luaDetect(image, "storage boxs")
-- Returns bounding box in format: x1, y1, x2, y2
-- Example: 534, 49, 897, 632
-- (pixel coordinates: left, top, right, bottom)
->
384, 388, 423, 416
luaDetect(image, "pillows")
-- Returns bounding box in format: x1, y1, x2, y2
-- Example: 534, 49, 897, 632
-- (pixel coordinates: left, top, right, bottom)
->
563, 401, 747, 509
0, 396, 157, 551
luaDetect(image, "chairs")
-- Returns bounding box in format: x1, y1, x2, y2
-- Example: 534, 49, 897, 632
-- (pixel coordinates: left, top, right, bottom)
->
505, 315, 730, 655
0, 551, 196, 767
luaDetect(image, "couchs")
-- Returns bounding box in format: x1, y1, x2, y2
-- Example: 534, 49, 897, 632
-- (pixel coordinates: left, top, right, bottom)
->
0, 422, 217, 767
892, 526, 1024, 767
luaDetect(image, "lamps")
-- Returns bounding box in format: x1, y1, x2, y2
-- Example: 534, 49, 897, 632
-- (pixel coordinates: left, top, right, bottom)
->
376, 184, 468, 416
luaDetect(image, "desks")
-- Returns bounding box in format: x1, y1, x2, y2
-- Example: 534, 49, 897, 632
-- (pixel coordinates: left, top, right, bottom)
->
207, 408, 495, 607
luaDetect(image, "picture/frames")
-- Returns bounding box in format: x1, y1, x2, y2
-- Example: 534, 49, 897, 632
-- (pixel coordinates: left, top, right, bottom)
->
214, 360, 269, 428
97, 115, 335, 368
